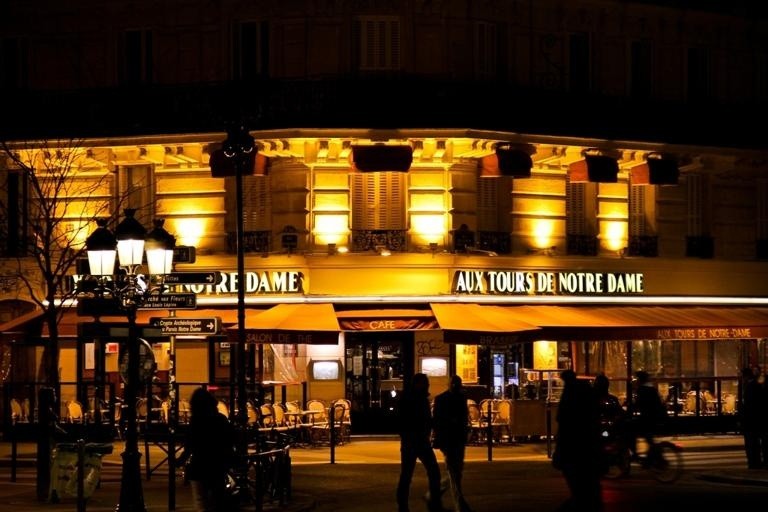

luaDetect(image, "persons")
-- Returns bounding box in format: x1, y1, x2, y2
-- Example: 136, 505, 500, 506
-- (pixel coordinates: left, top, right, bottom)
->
424, 376, 472, 511
553, 370, 666, 512
175, 387, 241, 511
736, 365, 768, 469
684, 386, 715, 416
395, 374, 447, 511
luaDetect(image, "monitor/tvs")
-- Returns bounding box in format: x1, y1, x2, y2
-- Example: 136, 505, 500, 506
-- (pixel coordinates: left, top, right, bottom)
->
418, 356, 450, 378
309, 360, 342, 382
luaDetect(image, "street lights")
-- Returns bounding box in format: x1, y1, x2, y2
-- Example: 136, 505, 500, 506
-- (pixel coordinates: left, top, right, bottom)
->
86, 209, 176, 512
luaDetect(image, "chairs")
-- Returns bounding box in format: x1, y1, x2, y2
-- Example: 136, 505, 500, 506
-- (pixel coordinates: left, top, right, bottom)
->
4, 396, 356, 452
686, 390, 739, 417
465, 399, 514, 446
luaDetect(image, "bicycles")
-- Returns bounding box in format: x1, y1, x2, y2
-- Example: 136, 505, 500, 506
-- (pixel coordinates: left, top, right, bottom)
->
599, 423, 683, 485
